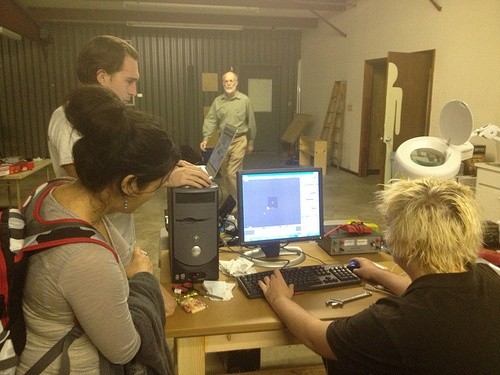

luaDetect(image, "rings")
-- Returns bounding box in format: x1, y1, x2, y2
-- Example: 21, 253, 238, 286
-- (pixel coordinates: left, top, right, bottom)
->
141, 250, 148, 256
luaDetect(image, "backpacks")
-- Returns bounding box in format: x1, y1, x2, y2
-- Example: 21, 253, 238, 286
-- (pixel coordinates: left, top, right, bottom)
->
0, 176, 120, 375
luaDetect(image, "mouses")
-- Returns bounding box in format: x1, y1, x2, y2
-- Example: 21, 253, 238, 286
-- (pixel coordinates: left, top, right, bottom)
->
347, 263, 361, 271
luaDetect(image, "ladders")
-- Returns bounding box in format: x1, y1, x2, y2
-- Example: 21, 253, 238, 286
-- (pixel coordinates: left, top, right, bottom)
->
319, 79, 348, 172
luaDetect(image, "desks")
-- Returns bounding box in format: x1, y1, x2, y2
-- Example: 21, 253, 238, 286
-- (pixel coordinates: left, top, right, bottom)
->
0, 158, 52, 208
160, 219, 405, 375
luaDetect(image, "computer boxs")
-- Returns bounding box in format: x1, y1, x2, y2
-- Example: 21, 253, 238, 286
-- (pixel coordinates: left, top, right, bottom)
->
164, 164, 219, 285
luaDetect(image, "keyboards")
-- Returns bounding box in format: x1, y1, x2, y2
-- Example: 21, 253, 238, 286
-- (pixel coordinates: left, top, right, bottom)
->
236, 263, 361, 300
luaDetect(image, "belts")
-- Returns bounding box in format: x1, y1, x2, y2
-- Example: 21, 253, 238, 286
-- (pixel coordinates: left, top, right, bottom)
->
235, 133, 246, 138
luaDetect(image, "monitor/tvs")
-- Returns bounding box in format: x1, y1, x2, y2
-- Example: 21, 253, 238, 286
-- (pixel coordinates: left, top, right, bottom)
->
235, 166, 324, 267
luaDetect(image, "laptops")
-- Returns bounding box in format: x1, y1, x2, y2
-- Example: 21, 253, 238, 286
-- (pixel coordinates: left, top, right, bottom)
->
196, 123, 239, 179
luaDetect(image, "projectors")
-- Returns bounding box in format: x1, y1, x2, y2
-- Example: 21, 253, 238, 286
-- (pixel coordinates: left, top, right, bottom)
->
318, 223, 380, 256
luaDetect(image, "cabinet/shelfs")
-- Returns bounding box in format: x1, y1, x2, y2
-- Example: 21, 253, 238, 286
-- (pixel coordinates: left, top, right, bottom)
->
300, 136, 327, 174
475, 161, 500, 224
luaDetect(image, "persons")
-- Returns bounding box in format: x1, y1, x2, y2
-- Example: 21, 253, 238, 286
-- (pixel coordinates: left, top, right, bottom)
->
200, 72, 256, 199
47, 34, 213, 268
257, 177, 500, 375
12, 86, 182, 375
